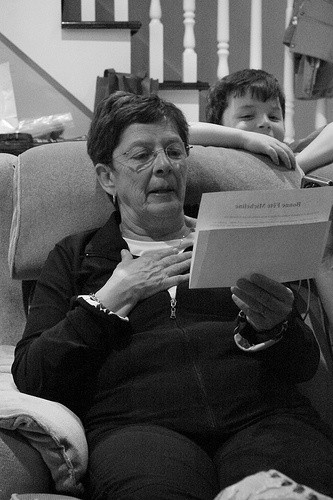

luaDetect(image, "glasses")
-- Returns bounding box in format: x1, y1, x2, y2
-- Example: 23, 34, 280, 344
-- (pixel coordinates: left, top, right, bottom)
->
113, 144, 194, 163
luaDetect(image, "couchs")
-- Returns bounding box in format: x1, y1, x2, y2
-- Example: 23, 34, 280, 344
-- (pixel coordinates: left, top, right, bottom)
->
1, 141, 333, 500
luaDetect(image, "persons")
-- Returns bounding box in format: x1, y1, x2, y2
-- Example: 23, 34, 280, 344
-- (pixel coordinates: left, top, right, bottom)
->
12, 91, 333, 500
187, 69, 333, 176
